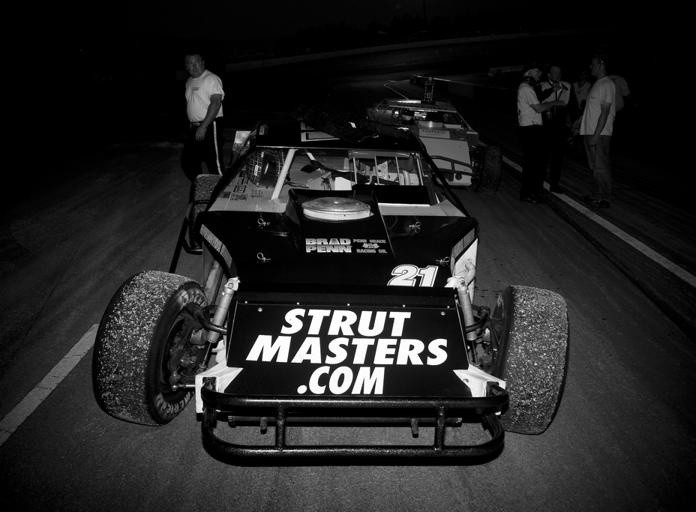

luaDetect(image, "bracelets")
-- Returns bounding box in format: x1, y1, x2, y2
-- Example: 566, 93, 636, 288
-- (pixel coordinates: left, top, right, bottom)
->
199, 125, 208, 130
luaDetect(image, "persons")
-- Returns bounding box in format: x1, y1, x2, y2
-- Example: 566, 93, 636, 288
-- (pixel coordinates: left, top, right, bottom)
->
514, 49, 633, 209
181, 49, 225, 182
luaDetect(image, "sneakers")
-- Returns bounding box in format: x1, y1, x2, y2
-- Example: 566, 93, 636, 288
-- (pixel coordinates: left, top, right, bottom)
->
518, 183, 611, 210
